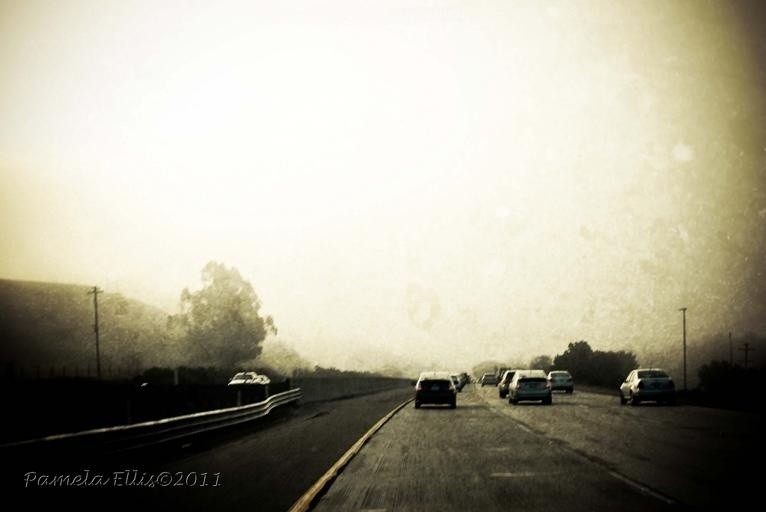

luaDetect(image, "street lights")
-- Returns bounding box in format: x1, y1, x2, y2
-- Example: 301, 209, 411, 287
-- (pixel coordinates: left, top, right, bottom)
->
677, 307, 688, 390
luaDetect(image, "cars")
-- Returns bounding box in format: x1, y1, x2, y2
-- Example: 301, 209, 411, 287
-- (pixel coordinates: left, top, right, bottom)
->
414, 371, 466, 410
227, 371, 271, 385
480, 368, 574, 404
620, 368, 675, 407
